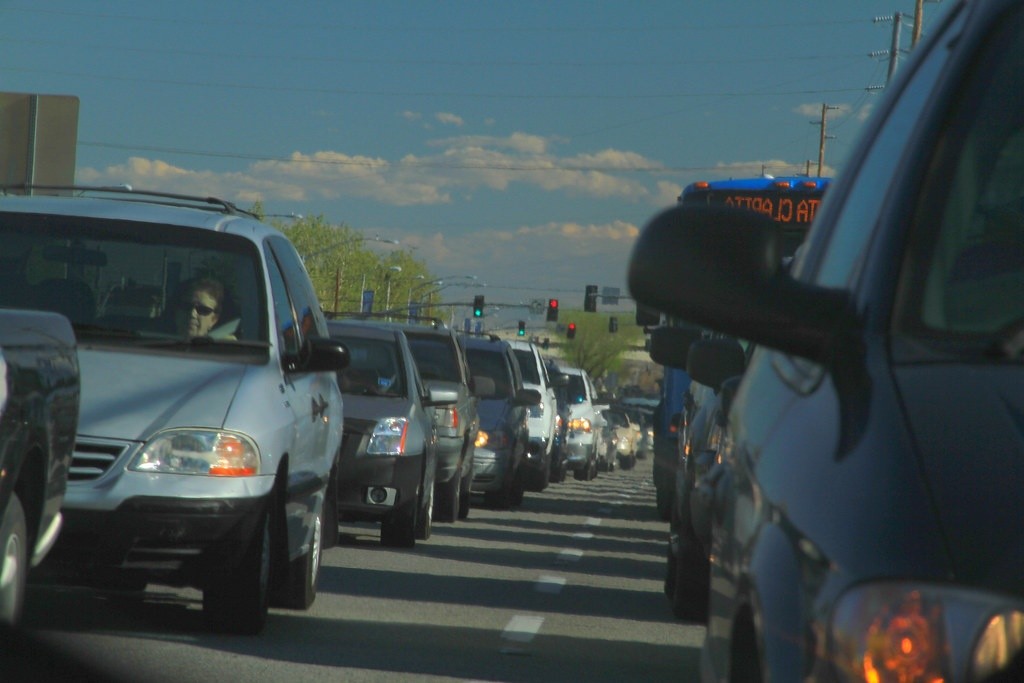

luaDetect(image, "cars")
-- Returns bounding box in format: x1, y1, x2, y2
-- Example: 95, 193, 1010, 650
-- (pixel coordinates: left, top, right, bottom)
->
456, 330, 541, 511
561, 366, 655, 473
320, 317, 457, 548
0, 309, 81, 668
355, 313, 480, 522
505, 339, 570, 490
629, 2, 1024, 679
666, 332, 746, 620
0, 185, 342, 638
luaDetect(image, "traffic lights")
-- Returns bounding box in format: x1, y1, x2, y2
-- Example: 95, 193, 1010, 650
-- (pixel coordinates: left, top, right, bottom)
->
518, 321, 525, 336
567, 322, 575, 336
474, 295, 484, 317
547, 299, 558, 320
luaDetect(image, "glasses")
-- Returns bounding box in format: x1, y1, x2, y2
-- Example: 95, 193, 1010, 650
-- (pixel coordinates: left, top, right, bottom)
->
178, 299, 212, 316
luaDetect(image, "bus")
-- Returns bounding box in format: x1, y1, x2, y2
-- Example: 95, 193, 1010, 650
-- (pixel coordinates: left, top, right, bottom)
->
655, 176, 830, 503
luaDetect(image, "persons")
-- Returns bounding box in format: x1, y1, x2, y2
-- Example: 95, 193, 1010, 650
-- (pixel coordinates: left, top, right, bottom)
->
155, 275, 237, 340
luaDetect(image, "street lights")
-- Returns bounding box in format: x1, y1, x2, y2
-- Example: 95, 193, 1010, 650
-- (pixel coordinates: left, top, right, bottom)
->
333, 266, 402, 322
419, 282, 488, 328
405, 273, 476, 324
303, 236, 400, 259
385, 275, 425, 318
259, 214, 304, 221
